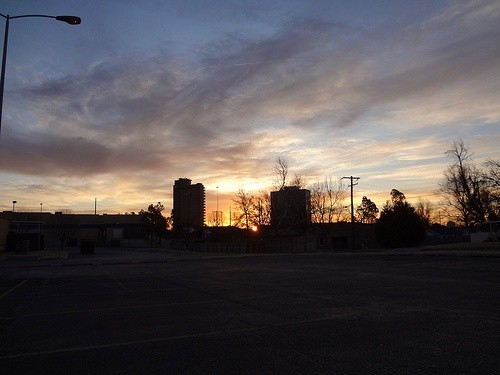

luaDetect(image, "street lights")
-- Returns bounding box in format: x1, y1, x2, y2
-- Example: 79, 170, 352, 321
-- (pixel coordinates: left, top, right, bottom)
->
0, 13, 81, 131
